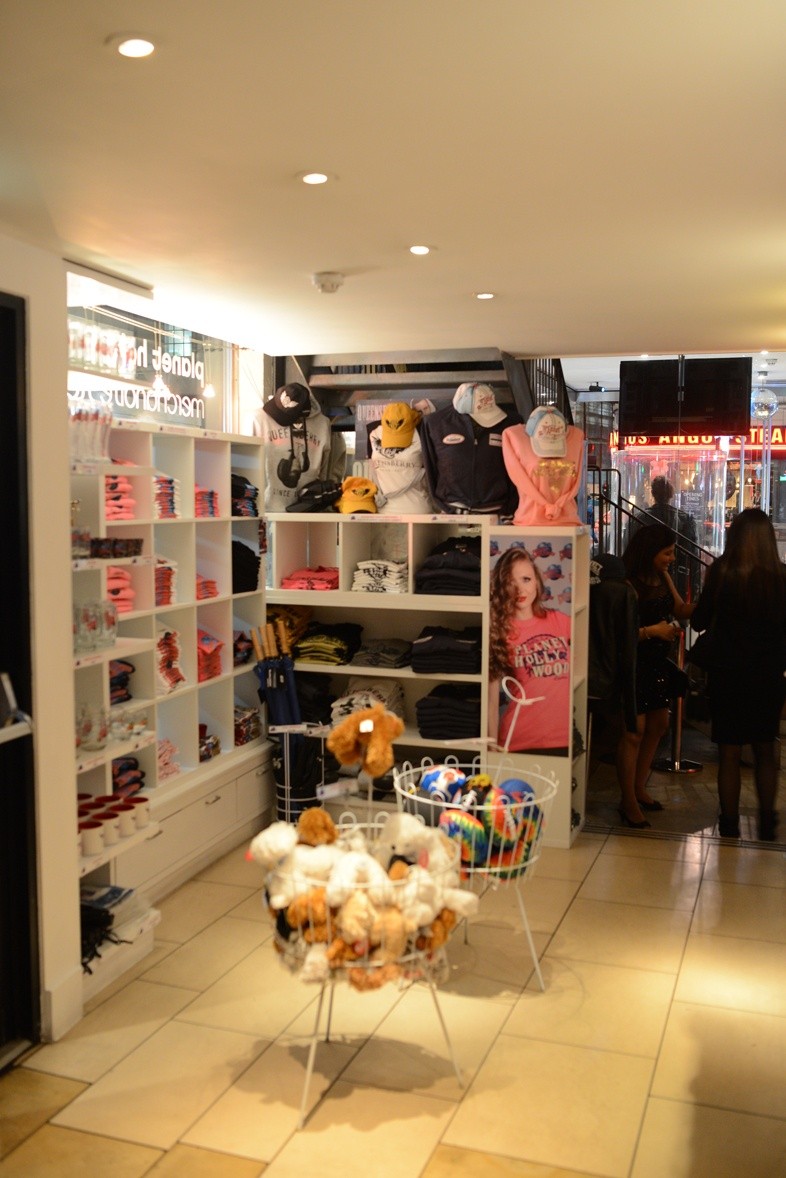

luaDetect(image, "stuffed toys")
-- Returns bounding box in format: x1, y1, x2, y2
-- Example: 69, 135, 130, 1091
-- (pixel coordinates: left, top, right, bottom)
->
326, 692, 405, 777
250, 807, 478, 990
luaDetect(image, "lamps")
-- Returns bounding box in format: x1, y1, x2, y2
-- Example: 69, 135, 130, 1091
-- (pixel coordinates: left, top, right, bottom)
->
589, 383, 605, 393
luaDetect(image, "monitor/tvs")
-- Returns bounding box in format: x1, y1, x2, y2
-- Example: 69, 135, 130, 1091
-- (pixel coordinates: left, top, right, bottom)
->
618, 356, 752, 437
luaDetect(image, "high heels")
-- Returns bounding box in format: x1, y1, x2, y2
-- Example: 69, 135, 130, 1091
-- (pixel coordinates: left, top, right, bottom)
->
618, 800, 651, 829
638, 795, 661, 811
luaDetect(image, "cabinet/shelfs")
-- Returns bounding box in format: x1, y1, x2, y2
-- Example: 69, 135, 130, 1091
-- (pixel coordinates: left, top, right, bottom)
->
261, 515, 591, 851
71, 418, 279, 1005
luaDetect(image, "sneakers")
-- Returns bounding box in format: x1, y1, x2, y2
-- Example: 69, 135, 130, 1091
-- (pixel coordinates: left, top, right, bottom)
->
718, 816, 741, 838
758, 816, 779, 842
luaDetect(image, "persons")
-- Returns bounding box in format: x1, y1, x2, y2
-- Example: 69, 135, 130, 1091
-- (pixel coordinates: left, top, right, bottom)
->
690, 508, 786, 840
489, 548, 572, 758
590, 476, 701, 829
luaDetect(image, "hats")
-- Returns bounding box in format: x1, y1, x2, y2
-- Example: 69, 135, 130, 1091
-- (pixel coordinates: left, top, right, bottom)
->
285, 480, 342, 513
380, 402, 421, 448
262, 381, 312, 427
453, 382, 507, 428
525, 406, 567, 457
335, 477, 379, 513
